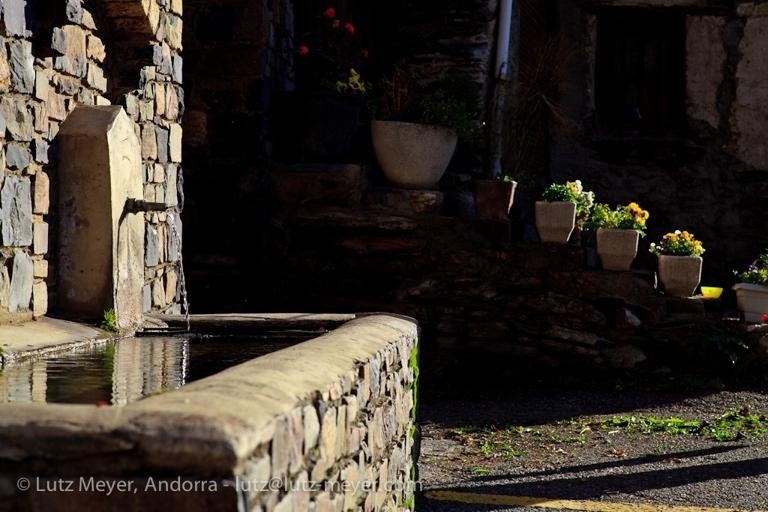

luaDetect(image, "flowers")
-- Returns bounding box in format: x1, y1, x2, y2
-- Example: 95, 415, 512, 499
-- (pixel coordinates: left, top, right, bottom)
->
648, 228, 714, 259
590, 198, 659, 232
539, 175, 596, 209
334, 62, 493, 133
286, 6, 376, 79
727, 241, 768, 286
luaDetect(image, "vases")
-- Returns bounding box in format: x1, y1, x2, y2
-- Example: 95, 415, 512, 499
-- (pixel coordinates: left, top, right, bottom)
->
468, 172, 523, 226
363, 110, 462, 195
653, 256, 707, 298
730, 286, 768, 331
590, 229, 647, 275
531, 200, 578, 249
